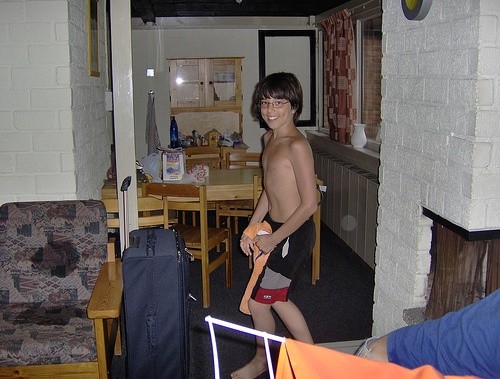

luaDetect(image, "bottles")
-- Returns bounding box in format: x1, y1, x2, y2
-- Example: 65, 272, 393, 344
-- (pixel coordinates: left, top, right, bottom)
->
351, 124, 367, 149
170, 116, 178, 148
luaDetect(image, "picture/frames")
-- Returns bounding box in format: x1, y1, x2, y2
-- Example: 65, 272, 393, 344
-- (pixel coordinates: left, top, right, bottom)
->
86, 0, 100, 77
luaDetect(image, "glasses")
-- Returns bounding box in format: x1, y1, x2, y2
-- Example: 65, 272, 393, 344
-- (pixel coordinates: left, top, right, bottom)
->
258, 100, 290, 108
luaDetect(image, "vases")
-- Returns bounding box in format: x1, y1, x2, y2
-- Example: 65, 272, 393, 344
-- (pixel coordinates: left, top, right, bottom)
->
352, 122, 367, 148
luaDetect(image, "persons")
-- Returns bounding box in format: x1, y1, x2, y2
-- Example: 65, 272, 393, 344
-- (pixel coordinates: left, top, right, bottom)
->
354, 288, 500, 379
230, 71, 319, 379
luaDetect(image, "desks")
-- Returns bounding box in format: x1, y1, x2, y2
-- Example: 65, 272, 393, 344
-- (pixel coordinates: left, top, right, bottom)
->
101, 168, 323, 285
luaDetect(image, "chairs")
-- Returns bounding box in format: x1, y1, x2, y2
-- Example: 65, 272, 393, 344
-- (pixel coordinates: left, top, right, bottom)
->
101, 146, 262, 309
0, 201, 121, 379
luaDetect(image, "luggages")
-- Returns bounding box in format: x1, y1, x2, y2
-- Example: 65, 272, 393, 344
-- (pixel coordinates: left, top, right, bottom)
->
121, 175, 196, 379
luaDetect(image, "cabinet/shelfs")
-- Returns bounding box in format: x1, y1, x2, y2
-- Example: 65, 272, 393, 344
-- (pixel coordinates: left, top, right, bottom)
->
167, 56, 249, 169
